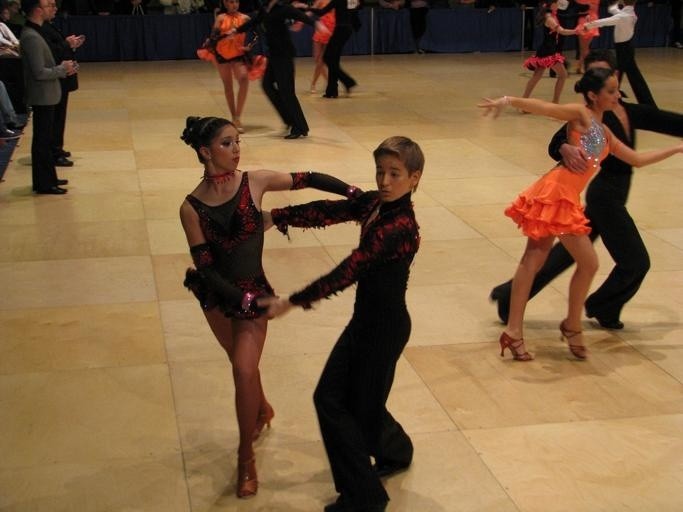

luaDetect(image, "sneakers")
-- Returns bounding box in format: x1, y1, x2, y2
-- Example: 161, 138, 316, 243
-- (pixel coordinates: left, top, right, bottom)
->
0, 129, 26, 141
6, 121, 27, 130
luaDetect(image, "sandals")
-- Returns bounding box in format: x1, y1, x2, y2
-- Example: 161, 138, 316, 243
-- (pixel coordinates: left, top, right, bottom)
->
237, 125, 245, 134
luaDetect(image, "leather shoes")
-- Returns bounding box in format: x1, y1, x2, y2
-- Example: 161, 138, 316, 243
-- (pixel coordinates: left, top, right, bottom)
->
323, 500, 390, 511
55, 156, 74, 167
584, 302, 625, 330
62, 149, 71, 157
56, 178, 68, 185
371, 455, 414, 481
284, 126, 309, 140
323, 93, 338, 98
310, 83, 316, 94
490, 286, 508, 325
35, 185, 68, 194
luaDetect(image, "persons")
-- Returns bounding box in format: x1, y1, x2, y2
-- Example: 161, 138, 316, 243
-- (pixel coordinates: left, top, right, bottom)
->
176, 114, 363, 499
520, 0, 656, 114
200, 1, 364, 139
0, 1, 84, 195
262, 136, 426, 511
488, 47, 683, 333
473, 67, 682, 362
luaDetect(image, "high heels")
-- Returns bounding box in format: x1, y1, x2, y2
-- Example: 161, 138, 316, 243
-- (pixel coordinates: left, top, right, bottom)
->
559, 320, 590, 360
237, 452, 259, 500
251, 406, 275, 442
497, 332, 532, 362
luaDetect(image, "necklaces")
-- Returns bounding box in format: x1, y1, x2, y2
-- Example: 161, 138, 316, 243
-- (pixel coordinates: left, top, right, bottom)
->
199, 169, 241, 186
